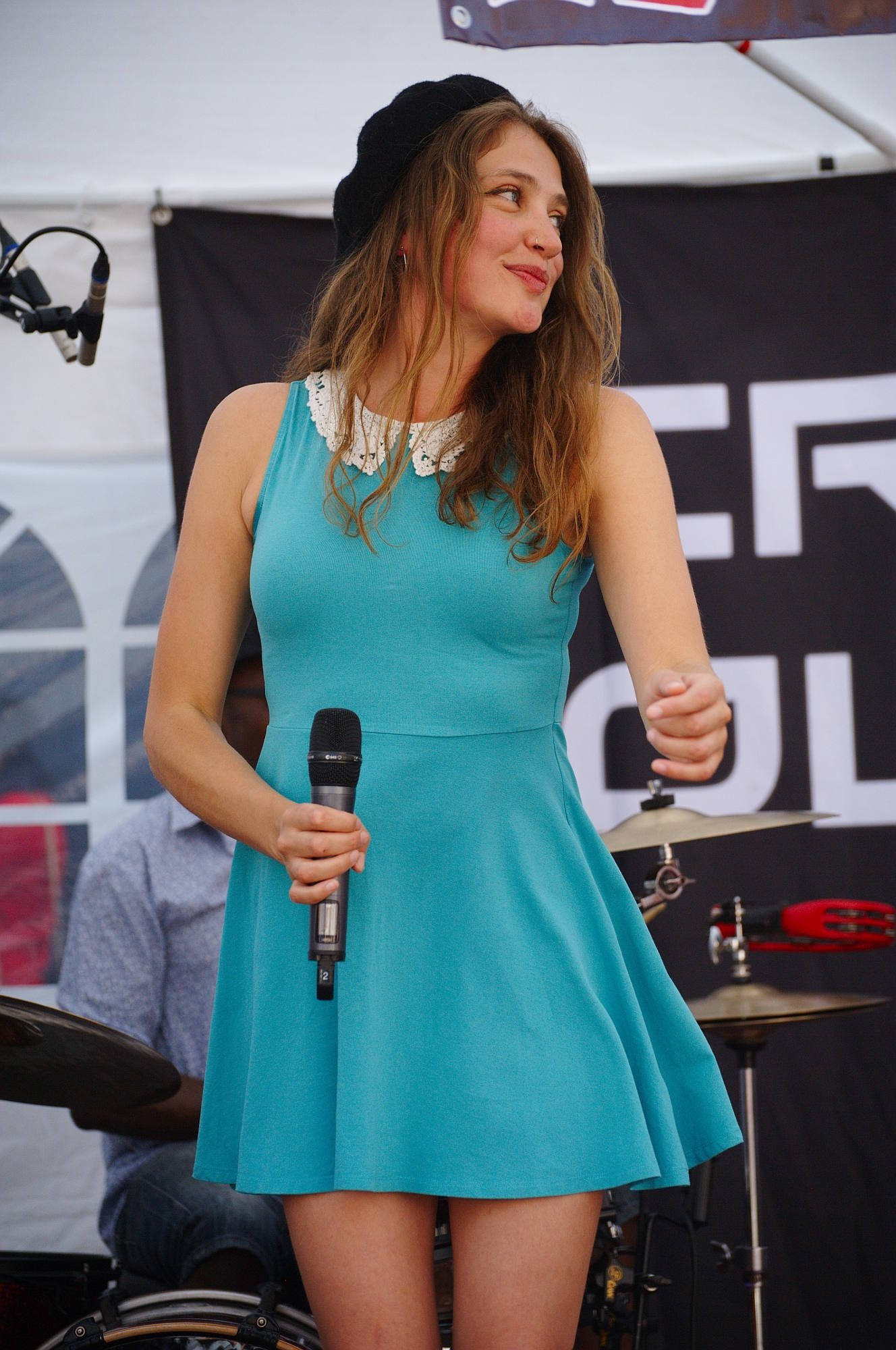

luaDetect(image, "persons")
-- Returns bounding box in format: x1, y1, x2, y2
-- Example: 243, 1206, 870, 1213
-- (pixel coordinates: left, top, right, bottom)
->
57, 616, 288, 1272
141, 74, 742, 1350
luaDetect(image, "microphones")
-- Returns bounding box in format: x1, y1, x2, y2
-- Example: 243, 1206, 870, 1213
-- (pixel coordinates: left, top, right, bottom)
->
77, 251, 112, 365
308, 709, 360, 998
1, 226, 79, 363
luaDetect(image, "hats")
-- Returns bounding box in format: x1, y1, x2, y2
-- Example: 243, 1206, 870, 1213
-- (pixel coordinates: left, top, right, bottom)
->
332, 75, 523, 273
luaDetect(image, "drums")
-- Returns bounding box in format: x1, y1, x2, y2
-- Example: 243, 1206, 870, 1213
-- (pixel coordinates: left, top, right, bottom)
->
432, 1189, 623, 1347
38, 1289, 323, 1350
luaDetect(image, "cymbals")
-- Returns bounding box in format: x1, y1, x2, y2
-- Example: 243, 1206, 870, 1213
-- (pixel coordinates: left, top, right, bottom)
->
599, 807, 840, 853
684, 982, 894, 1045
711, 895, 896, 955
0, 995, 183, 1112
